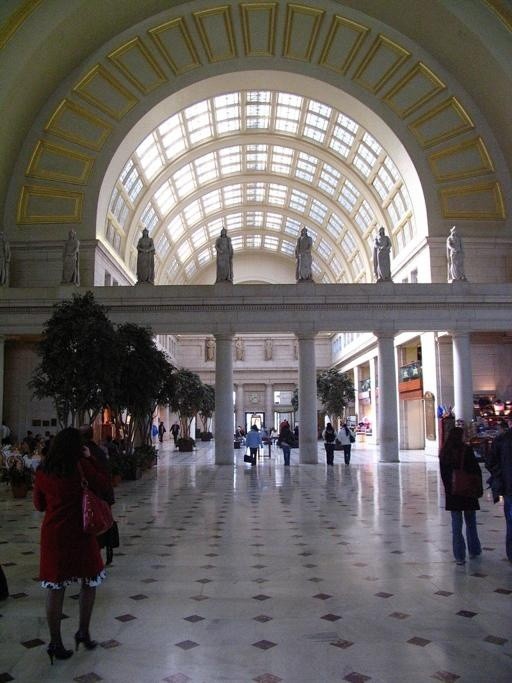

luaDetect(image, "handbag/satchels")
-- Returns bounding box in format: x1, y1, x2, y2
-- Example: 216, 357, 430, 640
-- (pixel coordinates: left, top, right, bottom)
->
451, 446, 481, 496
244, 454, 252, 462
350, 434, 355, 442
76, 459, 114, 535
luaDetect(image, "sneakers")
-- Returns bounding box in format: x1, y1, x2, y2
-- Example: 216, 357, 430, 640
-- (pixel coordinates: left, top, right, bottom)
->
456, 558, 465, 564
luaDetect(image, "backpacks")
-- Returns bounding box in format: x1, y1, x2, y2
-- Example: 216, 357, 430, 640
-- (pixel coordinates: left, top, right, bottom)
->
325, 430, 335, 441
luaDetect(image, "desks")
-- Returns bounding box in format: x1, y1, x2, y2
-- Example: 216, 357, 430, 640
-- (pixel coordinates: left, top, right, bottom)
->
2, 443, 42, 492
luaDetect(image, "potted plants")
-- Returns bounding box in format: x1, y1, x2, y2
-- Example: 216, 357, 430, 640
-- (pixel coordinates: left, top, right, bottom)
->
317, 367, 356, 450
4, 466, 31, 499
165, 367, 216, 454
26, 291, 174, 489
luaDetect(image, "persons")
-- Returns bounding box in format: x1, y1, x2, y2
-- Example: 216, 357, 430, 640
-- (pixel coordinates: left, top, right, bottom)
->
33, 426, 115, 664
137, 226, 155, 283
486, 410, 511, 560
169, 420, 180, 445
62, 229, 80, 283
294, 225, 313, 278
438, 426, 485, 564
337, 423, 355, 466
322, 421, 338, 465
80, 424, 120, 563
214, 226, 233, 281
238, 418, 298, 465
373, 225, 392, 279
444, 225, 466, 279
21, 429, 53, 457
103, 434, 136, 457
159, 420, 166, 441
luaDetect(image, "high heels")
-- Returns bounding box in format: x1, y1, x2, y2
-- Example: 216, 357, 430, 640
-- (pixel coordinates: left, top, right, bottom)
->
75, 629, 97, 649
48, 640, 73, 665
